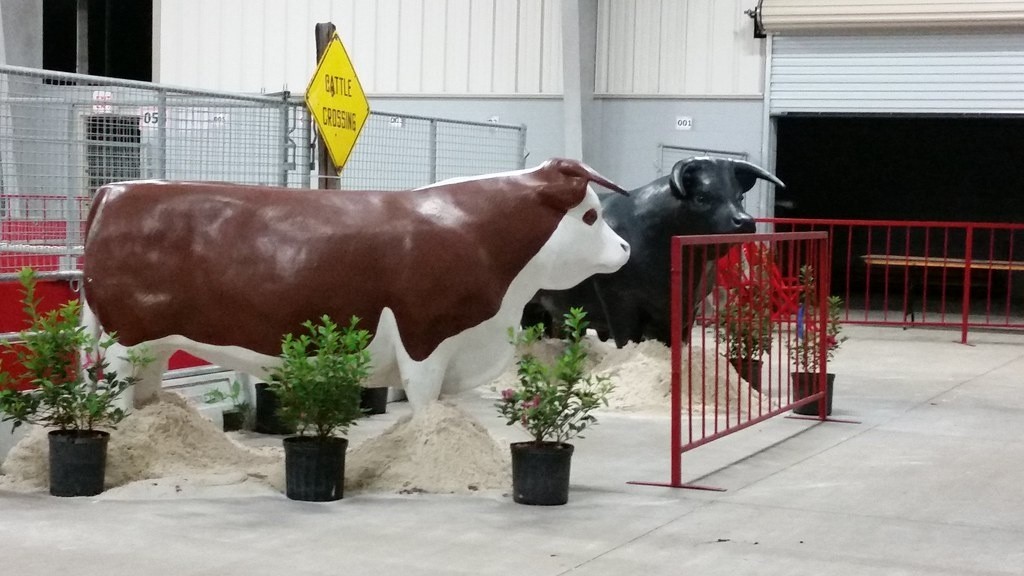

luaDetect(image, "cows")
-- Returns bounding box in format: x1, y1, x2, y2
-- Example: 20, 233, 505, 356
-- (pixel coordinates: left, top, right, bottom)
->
80, 158, 632, 410
521, 157, 786, 349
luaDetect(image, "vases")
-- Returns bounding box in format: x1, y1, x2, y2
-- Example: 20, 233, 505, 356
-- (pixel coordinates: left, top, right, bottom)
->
254, 383, 299, 435
509, 441, 574, 505
359, 385, 389, 415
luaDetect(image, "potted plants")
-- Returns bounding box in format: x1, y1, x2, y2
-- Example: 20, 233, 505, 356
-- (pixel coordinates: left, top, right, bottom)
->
781, 265, 848, 416
720, 246, 773, 392
0, 267, 157, 497
203, 379, 251, 431
261, 315, 376, 501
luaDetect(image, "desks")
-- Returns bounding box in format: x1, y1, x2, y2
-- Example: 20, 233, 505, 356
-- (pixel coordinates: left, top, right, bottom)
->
862, 254, 1024, 330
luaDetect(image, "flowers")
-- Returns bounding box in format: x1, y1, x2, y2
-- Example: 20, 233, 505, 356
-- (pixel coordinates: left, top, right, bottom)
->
488, 308, 618, 445
